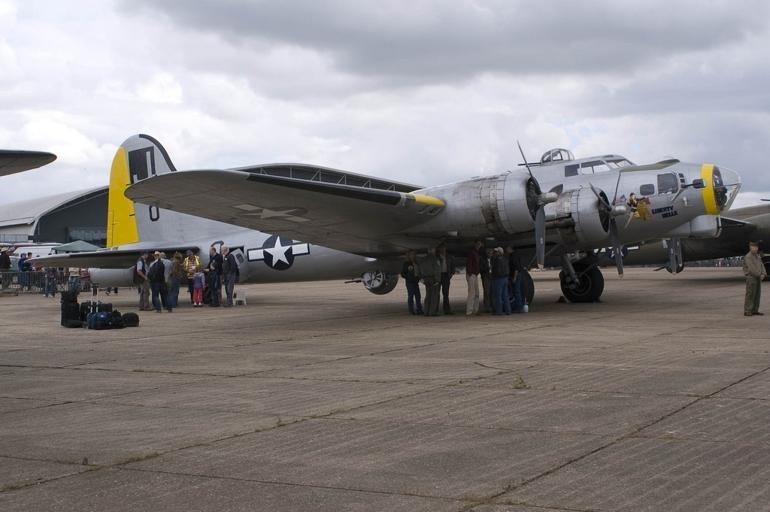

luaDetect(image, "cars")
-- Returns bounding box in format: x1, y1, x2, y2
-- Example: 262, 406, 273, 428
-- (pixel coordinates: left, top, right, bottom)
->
0, 256, 30, 286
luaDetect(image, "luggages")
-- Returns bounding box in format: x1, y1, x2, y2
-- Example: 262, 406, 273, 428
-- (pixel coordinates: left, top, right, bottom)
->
60, 290, 139, 329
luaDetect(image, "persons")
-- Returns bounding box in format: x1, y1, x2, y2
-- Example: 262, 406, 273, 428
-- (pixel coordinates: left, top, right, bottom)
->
743, 241, 767, 315
18, 251, 90, 298
401, 239, 527, 316
134, 244, 238, 312
624, 192, 647, 229
0, 250, 11, 288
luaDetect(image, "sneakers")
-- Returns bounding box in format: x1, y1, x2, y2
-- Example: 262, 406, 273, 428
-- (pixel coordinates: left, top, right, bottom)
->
137, 300, 233, 313
407, 306, 524, 316
743, 311, 764, 316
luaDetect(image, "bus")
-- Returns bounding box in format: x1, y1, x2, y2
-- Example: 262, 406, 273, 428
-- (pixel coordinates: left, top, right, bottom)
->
0, 241, 63, 257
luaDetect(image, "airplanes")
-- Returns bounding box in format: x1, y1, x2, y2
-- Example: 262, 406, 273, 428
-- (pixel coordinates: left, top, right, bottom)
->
23, 130, 769, 312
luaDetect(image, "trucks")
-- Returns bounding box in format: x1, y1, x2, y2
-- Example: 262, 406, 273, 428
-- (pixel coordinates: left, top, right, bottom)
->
7, 246, 70, 283
